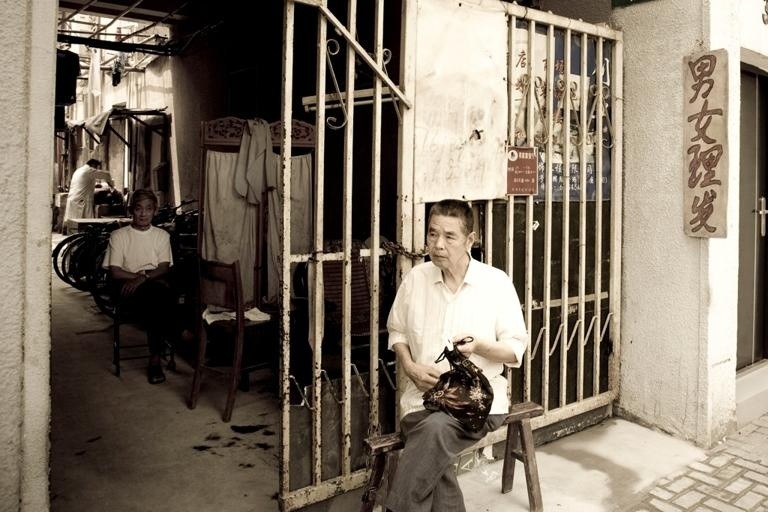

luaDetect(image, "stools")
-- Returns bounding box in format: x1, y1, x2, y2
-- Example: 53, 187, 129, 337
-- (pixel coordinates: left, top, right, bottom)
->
112, 300, 176, 380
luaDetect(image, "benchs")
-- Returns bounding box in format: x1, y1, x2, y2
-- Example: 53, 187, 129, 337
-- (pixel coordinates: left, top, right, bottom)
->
358, 399, 549, 511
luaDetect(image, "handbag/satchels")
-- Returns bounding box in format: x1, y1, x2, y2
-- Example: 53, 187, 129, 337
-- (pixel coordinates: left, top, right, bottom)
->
422, 336, 493, 432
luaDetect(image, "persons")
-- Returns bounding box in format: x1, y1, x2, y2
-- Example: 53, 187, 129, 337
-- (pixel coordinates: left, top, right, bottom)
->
62, 159, 114, 234
379, 199, 528, 512
102, 189, 193, 384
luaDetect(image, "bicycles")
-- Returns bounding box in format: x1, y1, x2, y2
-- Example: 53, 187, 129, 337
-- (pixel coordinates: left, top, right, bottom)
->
52, 188, 202, 334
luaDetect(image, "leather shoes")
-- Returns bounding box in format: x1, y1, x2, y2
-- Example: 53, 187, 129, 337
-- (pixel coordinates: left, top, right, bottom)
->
146, 364, 165, 384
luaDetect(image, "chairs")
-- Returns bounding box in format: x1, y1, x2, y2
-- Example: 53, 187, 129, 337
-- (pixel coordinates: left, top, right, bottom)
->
184, 253, 283, 421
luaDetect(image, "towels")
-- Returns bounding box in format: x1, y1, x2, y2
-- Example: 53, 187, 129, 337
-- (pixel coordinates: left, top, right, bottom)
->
201, 305, 272, 325
232, 120, 277, 206
